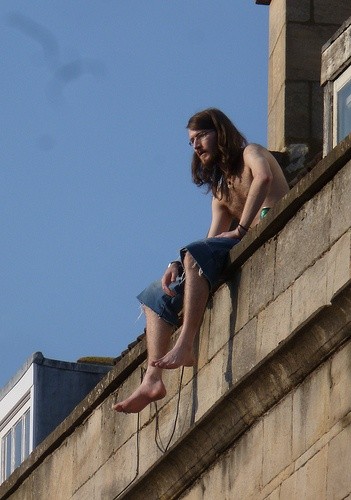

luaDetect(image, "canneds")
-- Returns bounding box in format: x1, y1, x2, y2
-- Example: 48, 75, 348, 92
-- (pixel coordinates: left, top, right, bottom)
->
260, 206, 272, 219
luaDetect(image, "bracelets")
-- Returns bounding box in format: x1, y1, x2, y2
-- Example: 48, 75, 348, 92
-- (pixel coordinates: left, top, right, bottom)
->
237, 223, 248, 236
166, 260, 183, 277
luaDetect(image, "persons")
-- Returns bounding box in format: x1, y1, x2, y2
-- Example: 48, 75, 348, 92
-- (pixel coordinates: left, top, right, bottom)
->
113, 107, 290, 413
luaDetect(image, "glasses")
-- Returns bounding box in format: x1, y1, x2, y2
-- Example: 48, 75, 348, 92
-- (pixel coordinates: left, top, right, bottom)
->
189, 128, 216, 146
